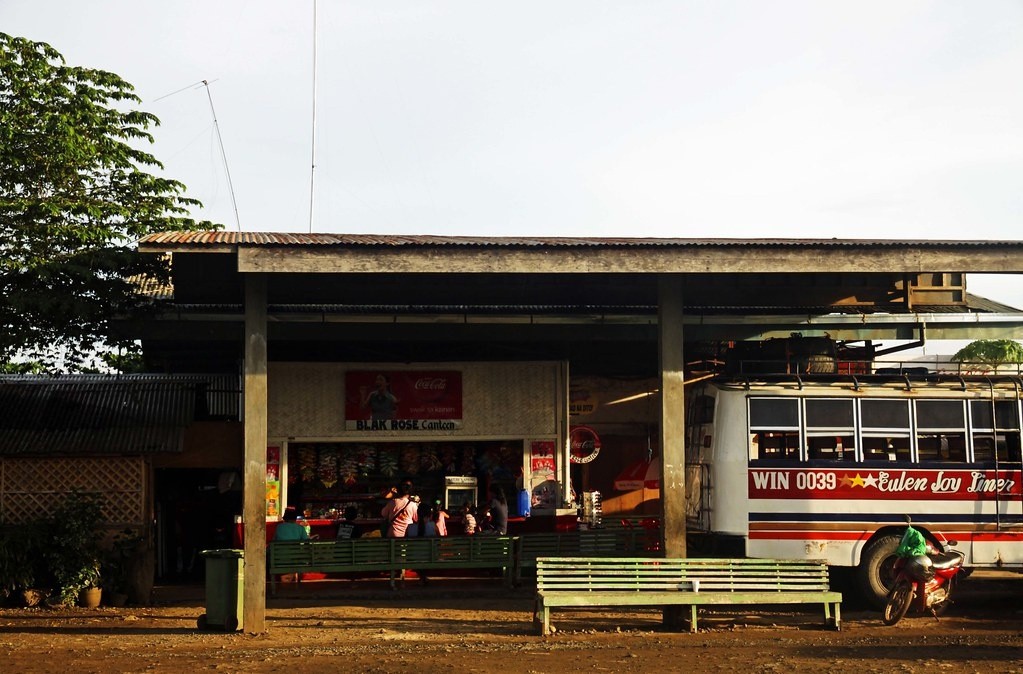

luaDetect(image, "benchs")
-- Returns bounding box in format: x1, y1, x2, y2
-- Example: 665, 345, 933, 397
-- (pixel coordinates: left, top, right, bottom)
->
582, 513, 668, 554
515, 529, 664, 590
269, 534, 515, 596
533, 556, 844, 639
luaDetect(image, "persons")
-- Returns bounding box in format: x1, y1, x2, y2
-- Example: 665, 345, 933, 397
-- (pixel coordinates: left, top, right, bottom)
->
434, 500, 450, 536
360, 374, 399, 419
382, 485, 420, 537
336, 506, 363, 540
405, 503, 441, 537
459, 496, 482, 534
276, 509, 310, 543
480, 482, 508, 535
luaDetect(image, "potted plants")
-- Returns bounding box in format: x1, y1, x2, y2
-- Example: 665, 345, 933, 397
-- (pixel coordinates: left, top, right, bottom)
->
1, 490, 155, 609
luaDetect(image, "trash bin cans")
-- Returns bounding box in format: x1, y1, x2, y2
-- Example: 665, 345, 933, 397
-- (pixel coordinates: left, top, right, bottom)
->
196, 548, 244, 630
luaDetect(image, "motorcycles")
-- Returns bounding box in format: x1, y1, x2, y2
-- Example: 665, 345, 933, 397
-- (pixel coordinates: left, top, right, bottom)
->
882, 514, 965, 626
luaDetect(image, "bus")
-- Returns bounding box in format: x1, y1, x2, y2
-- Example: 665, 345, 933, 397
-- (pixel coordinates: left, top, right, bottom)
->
685, 333, 1023, 612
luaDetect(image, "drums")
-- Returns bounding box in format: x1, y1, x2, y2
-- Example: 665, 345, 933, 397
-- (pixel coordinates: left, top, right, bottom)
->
791, 333, 838, 373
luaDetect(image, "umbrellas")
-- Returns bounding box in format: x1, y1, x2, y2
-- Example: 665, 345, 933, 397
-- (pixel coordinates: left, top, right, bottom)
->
612, 443, 660, 491
